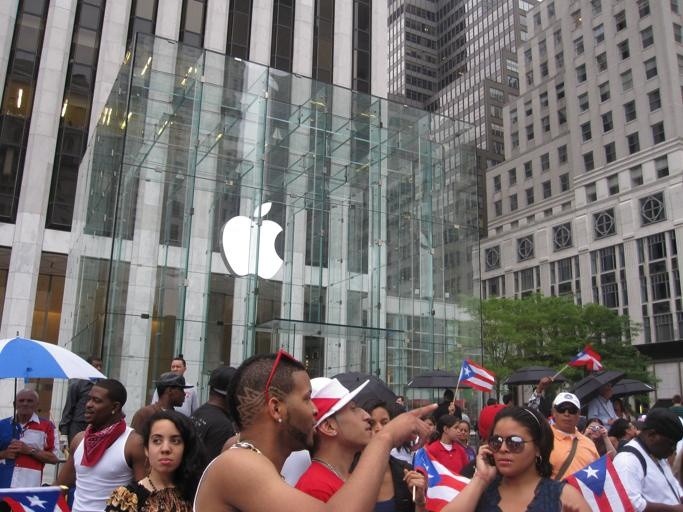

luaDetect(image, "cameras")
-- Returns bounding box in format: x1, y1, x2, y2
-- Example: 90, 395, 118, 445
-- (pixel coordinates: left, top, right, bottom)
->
592, 425, 601, 432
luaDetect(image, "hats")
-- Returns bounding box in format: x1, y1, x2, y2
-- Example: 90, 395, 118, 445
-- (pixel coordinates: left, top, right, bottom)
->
304, 376, 370, 432
632, 408, 683, 441
552, 392, 581, 409
207, 365, 241, 396
153, 372, 193, 389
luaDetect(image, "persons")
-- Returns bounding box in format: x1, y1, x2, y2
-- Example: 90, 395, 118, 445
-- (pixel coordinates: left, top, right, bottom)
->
0, 385, 58, 487
54, 355, 683, 512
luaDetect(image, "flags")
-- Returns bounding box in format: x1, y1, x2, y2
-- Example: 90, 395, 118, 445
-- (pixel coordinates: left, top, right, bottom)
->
565, 345, 602, 374
0, 488, 70, 511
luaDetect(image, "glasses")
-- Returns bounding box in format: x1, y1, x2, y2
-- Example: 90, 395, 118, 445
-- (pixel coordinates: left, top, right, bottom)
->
265, 347, 298, 407
556, 406, 577, 414
487, 435, 537, 454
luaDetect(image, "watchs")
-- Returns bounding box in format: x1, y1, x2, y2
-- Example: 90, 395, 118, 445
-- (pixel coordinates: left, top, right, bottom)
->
27, 447, 37, 457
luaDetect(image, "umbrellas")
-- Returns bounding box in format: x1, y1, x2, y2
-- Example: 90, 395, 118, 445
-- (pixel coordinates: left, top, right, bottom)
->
0, 331, 106, 435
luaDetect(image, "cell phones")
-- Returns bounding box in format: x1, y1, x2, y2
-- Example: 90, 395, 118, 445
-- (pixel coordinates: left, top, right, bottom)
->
487, 452, 495, 467
